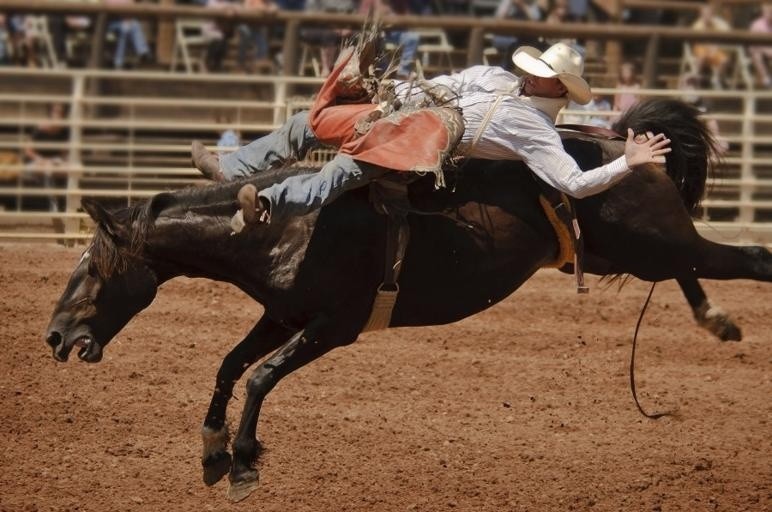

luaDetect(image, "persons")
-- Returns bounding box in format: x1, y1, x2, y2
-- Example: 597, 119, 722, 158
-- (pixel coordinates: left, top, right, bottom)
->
19, 98, 91, 212
1, 2, 772, 89
191, 44, 673, 236
610, 58, 642, 116
561, 91, 613, 130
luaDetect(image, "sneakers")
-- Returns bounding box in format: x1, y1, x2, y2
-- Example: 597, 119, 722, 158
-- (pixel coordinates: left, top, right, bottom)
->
190, 138, 229, 184
227, 183, 272, 238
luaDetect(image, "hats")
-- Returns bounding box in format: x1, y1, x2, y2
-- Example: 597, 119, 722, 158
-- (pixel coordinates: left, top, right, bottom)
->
511, 42, 593, 106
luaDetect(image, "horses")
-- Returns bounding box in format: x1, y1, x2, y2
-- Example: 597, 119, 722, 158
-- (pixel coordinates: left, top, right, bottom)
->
44, 96, 772, 504
